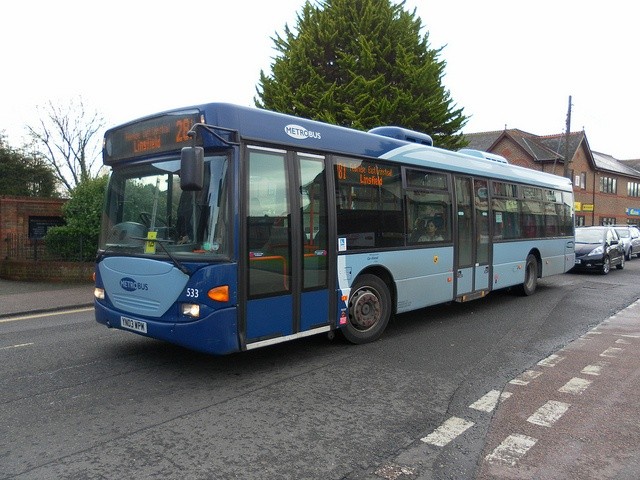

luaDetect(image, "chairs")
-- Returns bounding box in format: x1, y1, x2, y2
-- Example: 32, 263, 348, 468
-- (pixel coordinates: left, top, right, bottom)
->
176, 188, 200, 243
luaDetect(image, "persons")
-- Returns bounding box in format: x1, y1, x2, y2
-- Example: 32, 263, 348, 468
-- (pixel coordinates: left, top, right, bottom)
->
417, 220, 443, 241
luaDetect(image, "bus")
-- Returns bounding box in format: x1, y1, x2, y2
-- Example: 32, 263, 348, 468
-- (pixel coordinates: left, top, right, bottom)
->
95, 102, 577, 357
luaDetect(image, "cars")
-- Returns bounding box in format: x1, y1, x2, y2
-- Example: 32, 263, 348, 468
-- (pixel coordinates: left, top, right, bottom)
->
610, 226, 640, 260
574, 226, 625, 275
